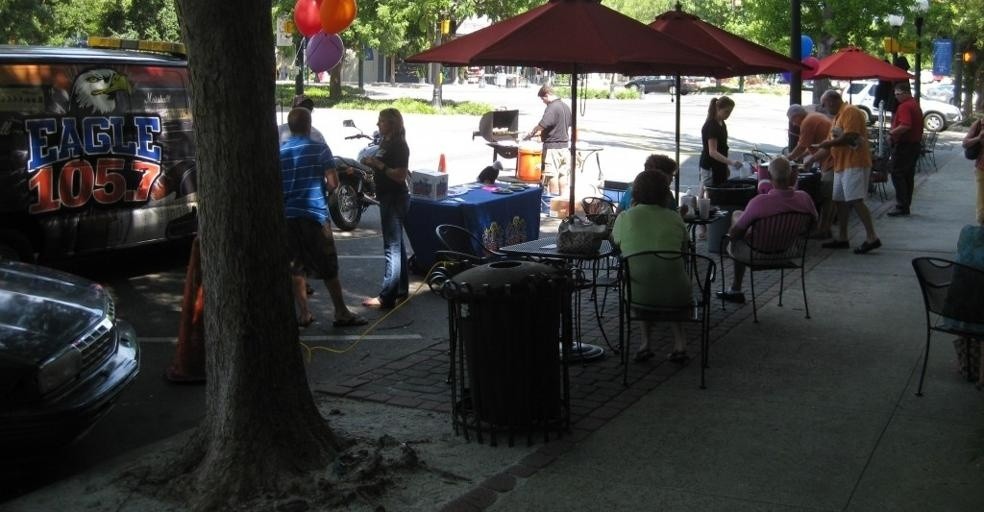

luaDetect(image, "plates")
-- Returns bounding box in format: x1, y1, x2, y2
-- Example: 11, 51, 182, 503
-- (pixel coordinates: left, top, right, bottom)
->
492, 184, 530, 194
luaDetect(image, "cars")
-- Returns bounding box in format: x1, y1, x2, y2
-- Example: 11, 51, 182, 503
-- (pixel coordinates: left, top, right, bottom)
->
0, 260, 140, 454
799, 64, 977, 130
625, 74, 699, 95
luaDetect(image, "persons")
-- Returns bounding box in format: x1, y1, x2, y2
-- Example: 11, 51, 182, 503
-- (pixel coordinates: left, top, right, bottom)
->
804, 88, 882, 254
522, 84, 572, 195
610, 168, 691, 362
716, 157, 818, 303
277, 93, 410, 327
698, 96, 743, 240
886, 81, 924, 215
615, 154, 688, 218
782, 103, 836, 238
874, 56, 910, 121
962, 99, 984, 225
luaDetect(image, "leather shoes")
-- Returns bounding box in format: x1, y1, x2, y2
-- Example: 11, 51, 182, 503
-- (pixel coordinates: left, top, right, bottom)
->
854, 239, 880, 253
822, 241, 849, 248
889, 209, 909, 215
716, 291, 742, 302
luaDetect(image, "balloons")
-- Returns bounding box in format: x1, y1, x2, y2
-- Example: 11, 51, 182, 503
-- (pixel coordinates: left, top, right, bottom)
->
292, 0, 358, 72
781, 34, 820, 83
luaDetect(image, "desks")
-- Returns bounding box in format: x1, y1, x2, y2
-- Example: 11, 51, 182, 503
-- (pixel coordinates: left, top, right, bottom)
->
505, 231, 627, 363
566, 145, 603, 179
678, 203, 734, 311
403, 178, 545, 273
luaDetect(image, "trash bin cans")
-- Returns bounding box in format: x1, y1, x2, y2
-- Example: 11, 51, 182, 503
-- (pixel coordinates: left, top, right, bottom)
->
427, 255, 586, 448
703, 182, 755, 254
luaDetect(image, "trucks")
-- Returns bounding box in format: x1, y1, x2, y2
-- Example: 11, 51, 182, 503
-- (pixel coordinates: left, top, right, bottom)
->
0, 31, 200, 271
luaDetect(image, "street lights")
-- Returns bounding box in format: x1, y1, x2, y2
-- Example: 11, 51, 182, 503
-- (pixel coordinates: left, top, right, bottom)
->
886, 2, 905, 69
909, 1, 927, 103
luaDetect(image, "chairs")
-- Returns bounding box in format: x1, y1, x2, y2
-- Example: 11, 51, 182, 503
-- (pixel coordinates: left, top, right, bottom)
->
433, 222, 507, 261
909, 254, 984, 404
726, 206, 820, 326
616, 251, 720, 391
580, 195, 623, 303
742, 125, 941, 239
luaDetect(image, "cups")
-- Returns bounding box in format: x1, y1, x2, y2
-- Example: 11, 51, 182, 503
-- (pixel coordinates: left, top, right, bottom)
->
698, 198, 710, 218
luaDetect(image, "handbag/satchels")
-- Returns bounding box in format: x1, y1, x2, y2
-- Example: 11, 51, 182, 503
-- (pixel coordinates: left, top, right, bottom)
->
955, 339, 979, 382
557, 215, 608, 253
965, 119, 981, 159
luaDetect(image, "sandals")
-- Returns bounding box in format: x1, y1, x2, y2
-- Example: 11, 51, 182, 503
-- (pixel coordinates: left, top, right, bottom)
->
668, 352, 688, 360
638, 351, 649, 360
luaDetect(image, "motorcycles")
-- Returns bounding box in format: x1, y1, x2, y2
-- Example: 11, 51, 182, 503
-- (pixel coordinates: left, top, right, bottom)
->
322, 113, 416, 234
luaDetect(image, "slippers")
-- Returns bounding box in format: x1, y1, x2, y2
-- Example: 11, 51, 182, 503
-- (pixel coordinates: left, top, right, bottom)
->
333, 314, 367, 325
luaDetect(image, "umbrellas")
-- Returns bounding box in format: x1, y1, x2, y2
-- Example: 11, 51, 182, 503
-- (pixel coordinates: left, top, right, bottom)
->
617, 0, 814, 207
815, 44, 917, 105
406, 0, 734, 226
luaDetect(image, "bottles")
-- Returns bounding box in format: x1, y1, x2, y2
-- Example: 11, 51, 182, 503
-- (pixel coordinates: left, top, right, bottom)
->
686, 187, 694, 195
681, 196, 697, 215
437, 153, 446, 173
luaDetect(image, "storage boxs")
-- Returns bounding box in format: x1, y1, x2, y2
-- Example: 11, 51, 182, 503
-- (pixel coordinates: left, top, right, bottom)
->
410, 169, 448, 203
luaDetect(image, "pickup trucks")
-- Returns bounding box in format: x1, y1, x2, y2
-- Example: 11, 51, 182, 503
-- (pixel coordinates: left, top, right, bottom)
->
675, 72, 718, 90
